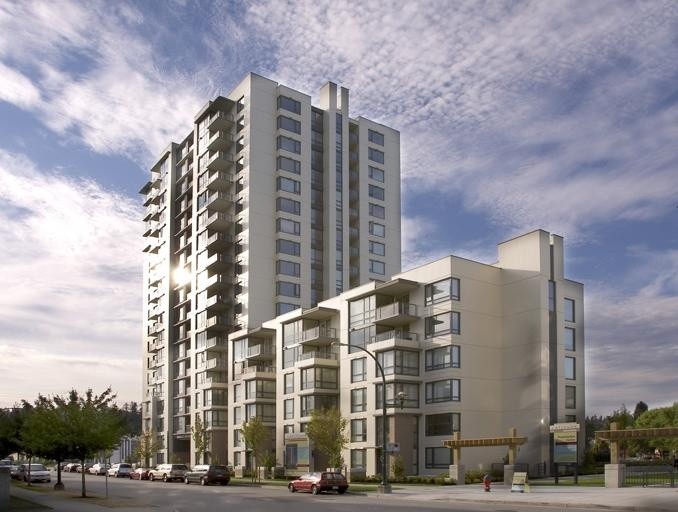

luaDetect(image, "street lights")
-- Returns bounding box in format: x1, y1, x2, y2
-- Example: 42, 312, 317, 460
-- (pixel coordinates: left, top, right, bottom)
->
329, 342, 392, 496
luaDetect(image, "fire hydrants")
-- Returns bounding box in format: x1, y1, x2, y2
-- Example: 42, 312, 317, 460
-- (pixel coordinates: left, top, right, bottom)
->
481, 474, 491, 493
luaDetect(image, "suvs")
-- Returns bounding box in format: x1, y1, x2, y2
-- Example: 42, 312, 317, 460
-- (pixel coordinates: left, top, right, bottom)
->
183, 464, 230, 486
148, 464, 189, 483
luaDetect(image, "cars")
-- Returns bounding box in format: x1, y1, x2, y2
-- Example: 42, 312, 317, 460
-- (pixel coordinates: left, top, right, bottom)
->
53, 463, 152, 480
287, 472, 347, 496
0, 455, 51, 484
